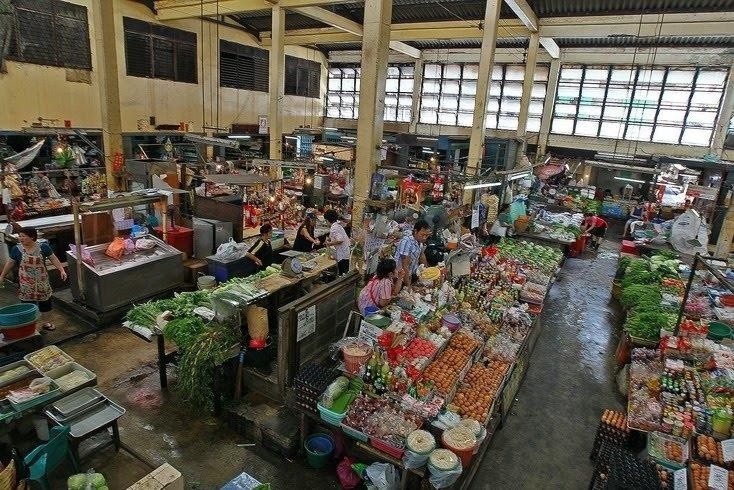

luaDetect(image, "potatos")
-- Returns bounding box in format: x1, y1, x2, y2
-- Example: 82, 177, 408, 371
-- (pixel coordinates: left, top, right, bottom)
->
423, 334, 510, 422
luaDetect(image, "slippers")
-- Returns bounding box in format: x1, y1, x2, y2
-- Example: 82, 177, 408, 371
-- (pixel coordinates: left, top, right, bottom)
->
42, 322, 56, 331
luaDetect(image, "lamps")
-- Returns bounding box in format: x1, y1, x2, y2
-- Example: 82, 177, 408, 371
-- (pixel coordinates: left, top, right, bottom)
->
184, 0, 667, 190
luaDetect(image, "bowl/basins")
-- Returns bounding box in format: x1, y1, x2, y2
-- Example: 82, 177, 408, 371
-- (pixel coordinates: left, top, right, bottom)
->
0, 302, 39, 339
706, 321, 733, 340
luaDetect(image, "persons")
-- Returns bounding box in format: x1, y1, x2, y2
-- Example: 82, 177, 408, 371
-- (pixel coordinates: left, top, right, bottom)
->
293, 214, 321, 252
355, 255, 407, 316
0, 226, 67, 331
479, 192, 491, 236
245, 223, 272, 271
32, 166, 51, 199
394, 219, 433, 286
323, 208, 349, 275
497, 203, 513, 229
581, 211, 610, 250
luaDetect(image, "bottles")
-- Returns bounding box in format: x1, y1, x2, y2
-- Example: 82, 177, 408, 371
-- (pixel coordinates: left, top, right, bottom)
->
362, 354, 407, 395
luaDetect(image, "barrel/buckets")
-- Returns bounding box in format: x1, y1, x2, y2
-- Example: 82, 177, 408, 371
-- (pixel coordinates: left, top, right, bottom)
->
197, 275, 215, 289
707, 321, 733, 345
303, 433, 334, 469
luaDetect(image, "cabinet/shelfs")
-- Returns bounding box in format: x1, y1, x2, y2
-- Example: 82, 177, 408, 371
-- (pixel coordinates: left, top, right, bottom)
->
0, 195, 575, 490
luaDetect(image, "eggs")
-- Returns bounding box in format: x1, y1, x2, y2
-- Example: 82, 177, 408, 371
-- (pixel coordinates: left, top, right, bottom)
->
600, 412, 734, 490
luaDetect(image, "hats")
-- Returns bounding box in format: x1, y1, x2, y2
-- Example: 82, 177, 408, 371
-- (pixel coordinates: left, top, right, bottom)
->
307, 213, 319, 221
